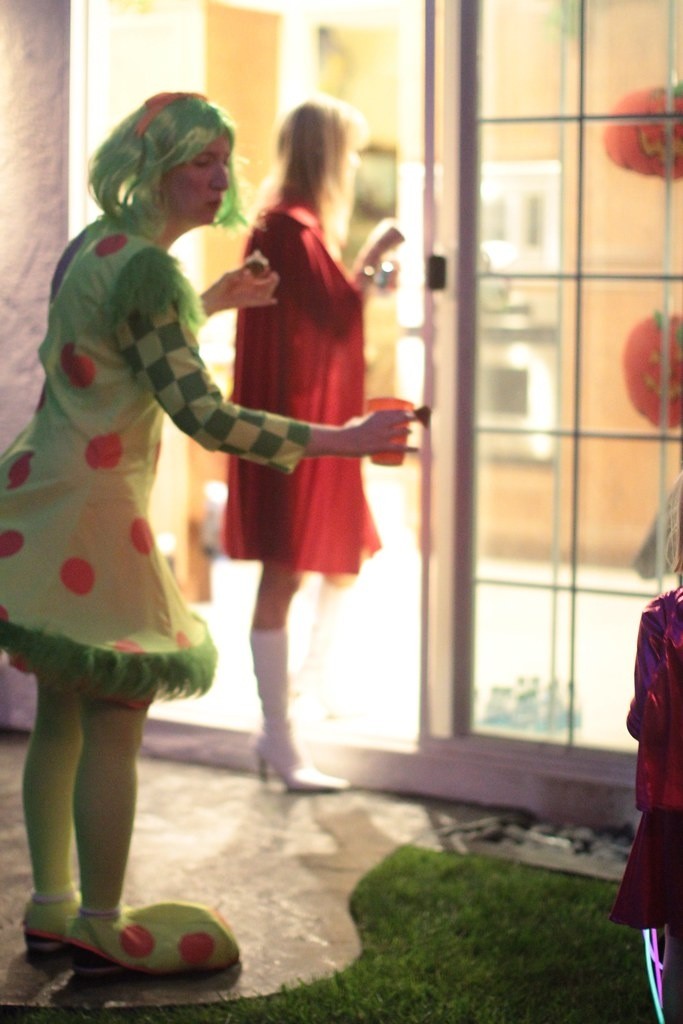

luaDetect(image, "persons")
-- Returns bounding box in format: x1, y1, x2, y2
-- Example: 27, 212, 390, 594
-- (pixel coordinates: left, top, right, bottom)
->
0, 92, 422, 978
222, 94, 406, 793
608, 472, 683, 1023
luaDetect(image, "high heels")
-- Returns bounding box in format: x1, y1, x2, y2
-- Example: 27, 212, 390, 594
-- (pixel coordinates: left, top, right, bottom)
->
256, 725, 352, 792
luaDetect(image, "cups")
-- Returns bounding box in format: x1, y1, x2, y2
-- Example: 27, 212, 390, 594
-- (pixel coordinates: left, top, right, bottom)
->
367, 396, 414, 465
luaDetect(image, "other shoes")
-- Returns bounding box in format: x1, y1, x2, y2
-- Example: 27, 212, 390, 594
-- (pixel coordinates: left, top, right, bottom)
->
22, 932, 67, 961
67, 942, 164, 978
